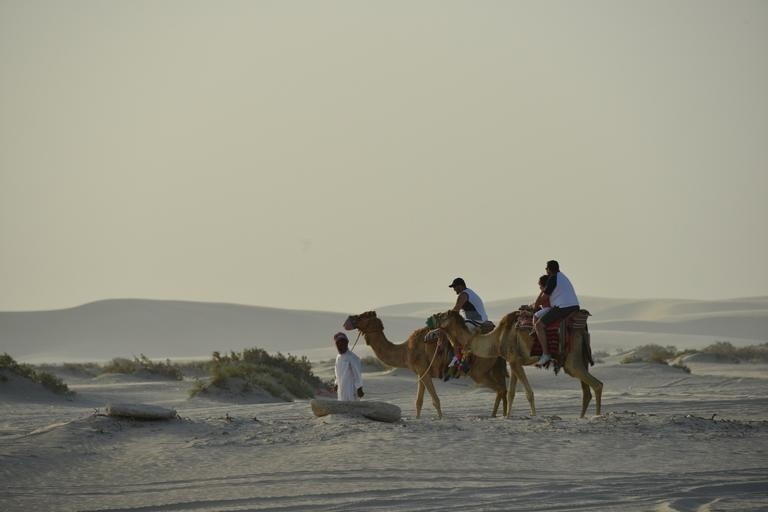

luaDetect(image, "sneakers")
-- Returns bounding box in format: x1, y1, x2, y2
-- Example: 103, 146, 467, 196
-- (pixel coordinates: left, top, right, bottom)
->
528, 327, 536, 336
535, 352, 551, 366
447, 354, 459, 368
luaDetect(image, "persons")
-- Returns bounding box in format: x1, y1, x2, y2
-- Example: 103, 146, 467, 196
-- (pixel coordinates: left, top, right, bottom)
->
528, 258, 580, 368
330, 331, 365, 402
527, 274, 553, 335
445, 276, 489, 368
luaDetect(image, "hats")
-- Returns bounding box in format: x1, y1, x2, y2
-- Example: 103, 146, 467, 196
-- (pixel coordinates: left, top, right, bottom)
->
449, 278, 465, 287
334, 332, 348, 344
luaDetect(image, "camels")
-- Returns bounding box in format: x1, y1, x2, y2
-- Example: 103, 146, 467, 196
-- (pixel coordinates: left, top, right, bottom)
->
342, 310, 510, 419
425, 302, 604, 419
